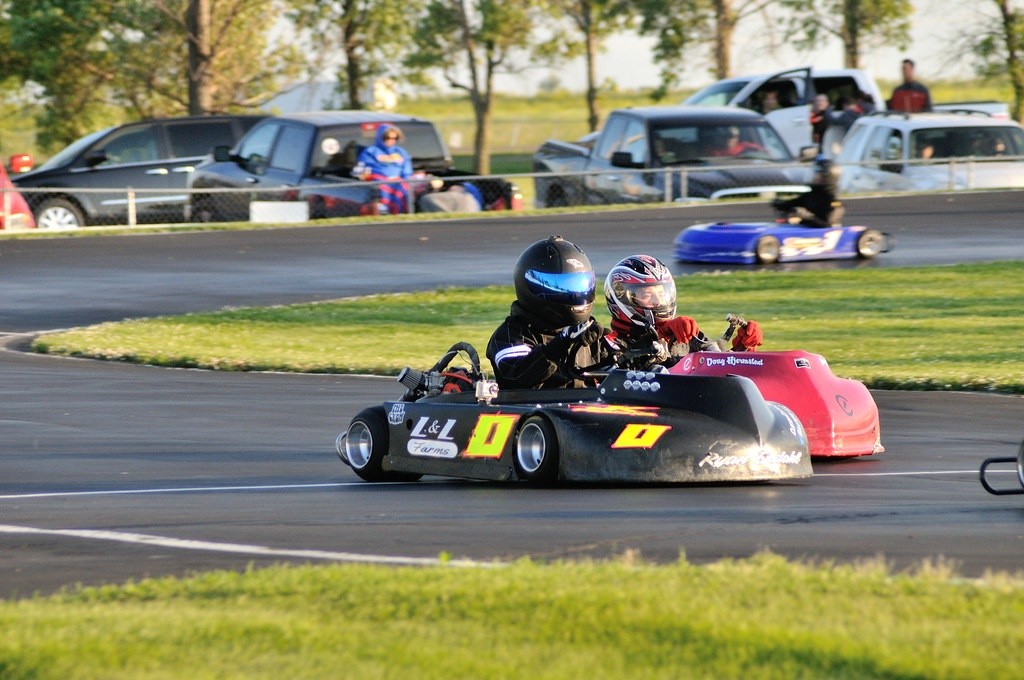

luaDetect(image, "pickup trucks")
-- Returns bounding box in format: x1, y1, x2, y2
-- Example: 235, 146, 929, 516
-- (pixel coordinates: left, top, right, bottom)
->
532, 102, 820, 205
187, 107, 524, 215
681, 67, 1011, 188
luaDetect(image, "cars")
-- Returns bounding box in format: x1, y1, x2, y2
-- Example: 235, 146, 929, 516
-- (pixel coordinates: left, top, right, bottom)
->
829, 113, 1024, 199
0, 148, 38, 231
10, 110, 279, 229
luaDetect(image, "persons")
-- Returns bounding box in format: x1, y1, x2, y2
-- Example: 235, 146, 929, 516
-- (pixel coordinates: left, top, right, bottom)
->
769, 159, 846, 228
487, 234, 659, 390
652, 59, 1006, 165
351, 124, 484, 216
604, 255, 763, 369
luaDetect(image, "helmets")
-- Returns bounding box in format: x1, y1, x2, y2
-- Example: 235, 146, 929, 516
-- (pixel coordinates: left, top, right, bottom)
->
511, 236, 595, 334
604, 255, 676, 333
813, 157, 840, 187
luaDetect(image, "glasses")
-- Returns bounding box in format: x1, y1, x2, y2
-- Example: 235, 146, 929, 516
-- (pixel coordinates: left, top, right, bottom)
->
383, 135, 399, 141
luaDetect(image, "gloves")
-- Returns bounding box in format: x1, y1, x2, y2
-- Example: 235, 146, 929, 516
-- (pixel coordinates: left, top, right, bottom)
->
770, 199, 785, 209
792, 207, 814, 221
570, 317, 611, 347
732, 320, 763, 352
612, 329, 656, 352
657, 316, 699, 344
